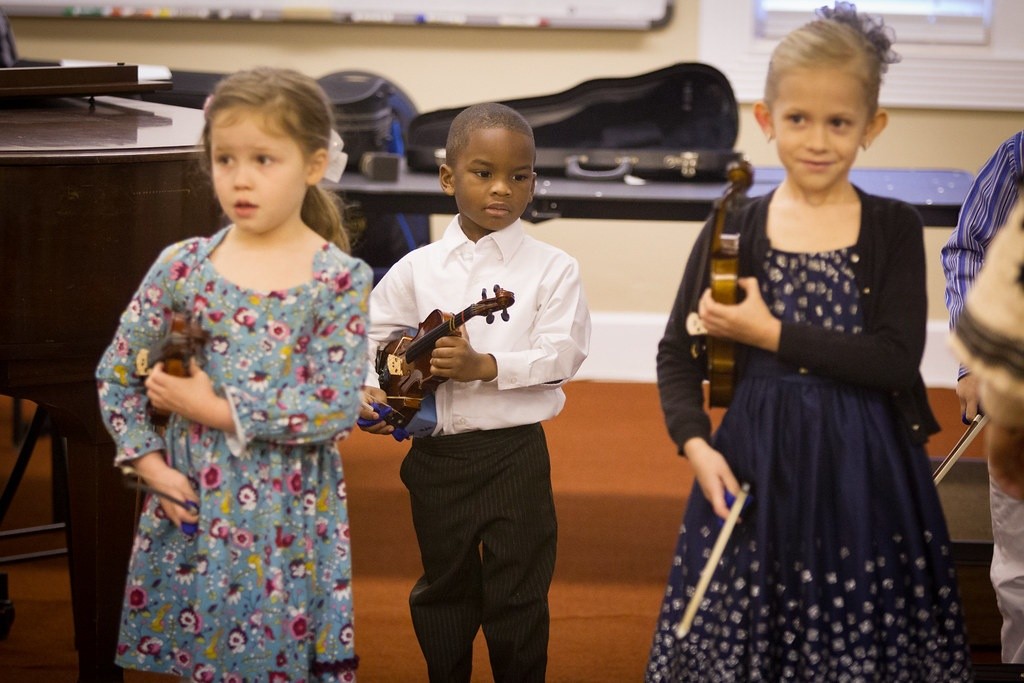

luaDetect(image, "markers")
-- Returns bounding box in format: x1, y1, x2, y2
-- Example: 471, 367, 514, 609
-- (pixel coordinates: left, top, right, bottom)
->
62, 6, 549, 27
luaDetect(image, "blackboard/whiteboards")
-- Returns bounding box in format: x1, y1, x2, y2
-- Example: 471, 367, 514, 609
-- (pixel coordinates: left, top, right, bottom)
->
1, 1, 673, 31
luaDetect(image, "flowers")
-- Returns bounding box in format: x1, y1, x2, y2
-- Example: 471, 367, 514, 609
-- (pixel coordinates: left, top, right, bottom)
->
815, 1, 901, 75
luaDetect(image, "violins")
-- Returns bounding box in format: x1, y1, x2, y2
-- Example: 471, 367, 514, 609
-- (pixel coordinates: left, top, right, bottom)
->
705, 158, 756, 409
150, 308, 211, 428
373, 283, 516, 431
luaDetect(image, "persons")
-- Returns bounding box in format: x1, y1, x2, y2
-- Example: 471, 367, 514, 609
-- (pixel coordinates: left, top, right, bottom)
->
644, 2, 972, 683
359, 102, 592, 683
96, 69, 373, 683
941, 131, 1024, 665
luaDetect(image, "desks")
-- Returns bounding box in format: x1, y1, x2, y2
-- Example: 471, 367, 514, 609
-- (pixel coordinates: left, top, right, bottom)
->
339, 166, 982, 223
